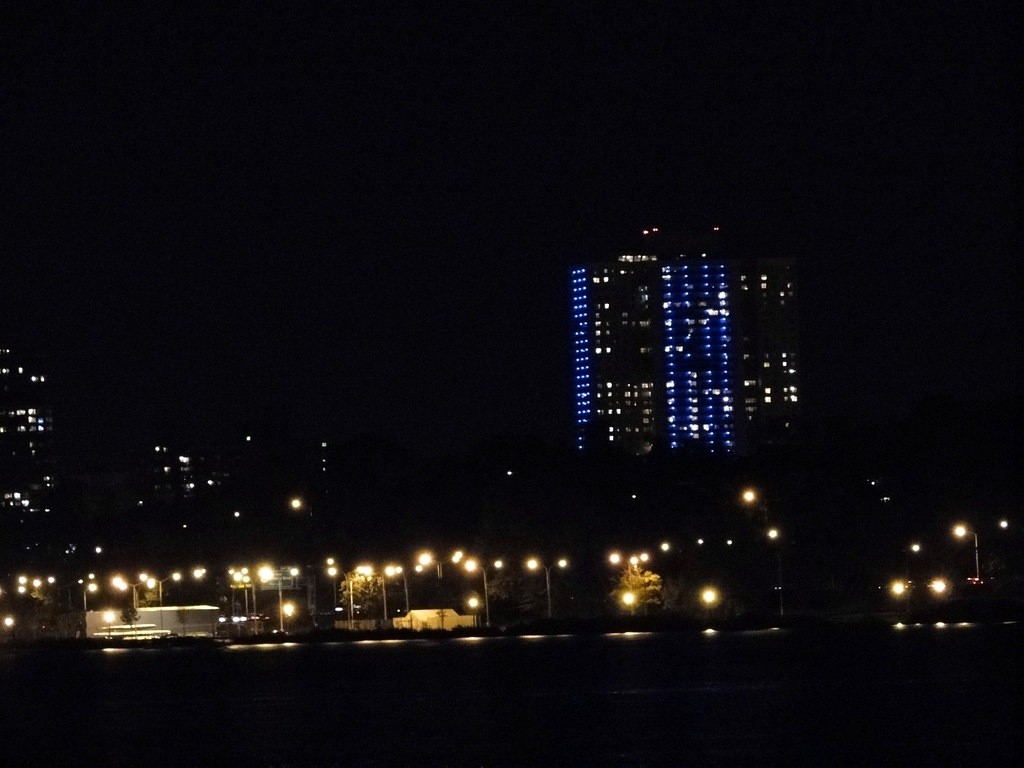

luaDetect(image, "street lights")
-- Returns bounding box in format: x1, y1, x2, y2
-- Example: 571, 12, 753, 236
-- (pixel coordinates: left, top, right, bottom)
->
951, 523, 979, 580
905, 543, 921, 610
228, 562, 302, 636
146, 572, 183, 630
414, 549, 465, 579
323, 557, 424, 629
110, 570, 149, 608
463, 558, 504, 627
524, 556, 569, 619
